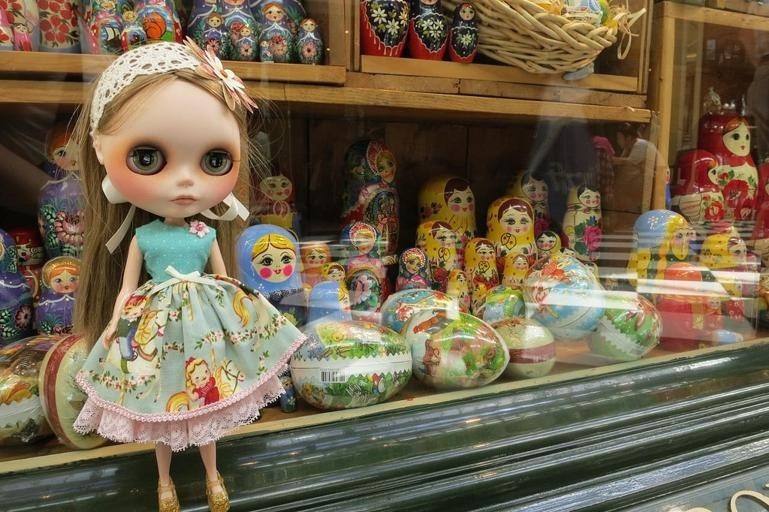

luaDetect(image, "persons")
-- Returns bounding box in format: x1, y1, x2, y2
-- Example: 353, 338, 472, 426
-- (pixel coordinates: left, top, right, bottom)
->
64, 40, 302, 511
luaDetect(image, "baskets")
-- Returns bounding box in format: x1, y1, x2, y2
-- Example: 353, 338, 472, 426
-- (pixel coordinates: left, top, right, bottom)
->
438, 1, 647, 75
